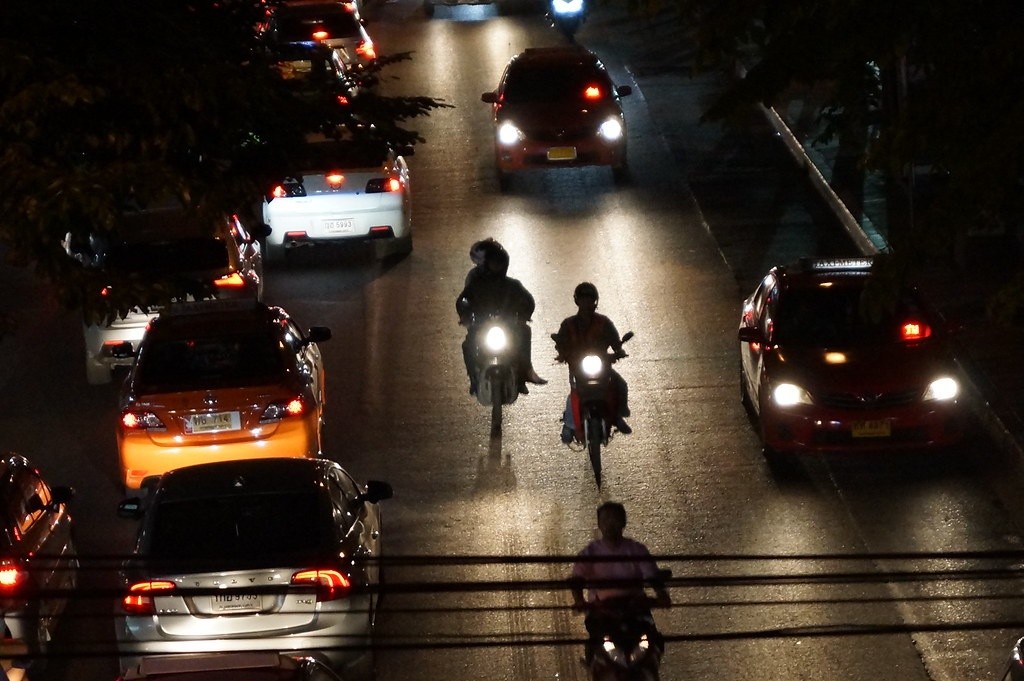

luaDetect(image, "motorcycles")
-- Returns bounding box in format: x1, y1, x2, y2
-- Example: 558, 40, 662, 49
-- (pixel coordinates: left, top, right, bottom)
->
546, 0, 588, 37
457, 307, 524, 434
550, 332, 636, 472
564, 567, 673, 681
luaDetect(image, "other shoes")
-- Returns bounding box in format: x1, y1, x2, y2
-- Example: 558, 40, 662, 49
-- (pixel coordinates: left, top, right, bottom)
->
615, 419, 632, 434
469, 380, 476, 395
527, 372, 547, 385
561, 425, 574, 444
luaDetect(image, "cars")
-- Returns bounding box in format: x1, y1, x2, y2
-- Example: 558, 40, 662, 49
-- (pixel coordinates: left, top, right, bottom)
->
733, 256, 972, 466
98, 303, 333, 518
115, 456, 391, 681
481, 46, 632, 181
0, 445, 91, 659
2, 1, 499, 392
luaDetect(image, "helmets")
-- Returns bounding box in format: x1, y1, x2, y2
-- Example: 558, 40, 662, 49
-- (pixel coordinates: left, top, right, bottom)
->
485, 247, 509, 275
574, 282, 598, 309
470, 238, 501, 265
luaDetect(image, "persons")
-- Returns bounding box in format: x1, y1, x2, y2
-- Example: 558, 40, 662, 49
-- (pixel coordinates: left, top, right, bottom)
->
566, 502, 673, 659
553, 280, 632, 440
451, 236, 547, 394
